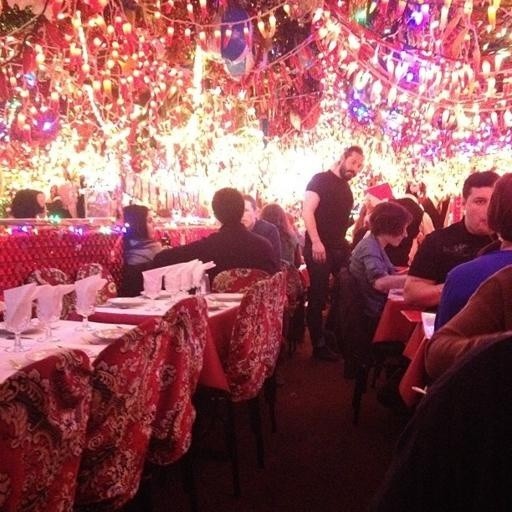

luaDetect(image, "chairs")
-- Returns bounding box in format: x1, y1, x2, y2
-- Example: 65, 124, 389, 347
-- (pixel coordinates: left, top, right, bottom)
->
0, 258, 306, 512
337, 265, 406, 413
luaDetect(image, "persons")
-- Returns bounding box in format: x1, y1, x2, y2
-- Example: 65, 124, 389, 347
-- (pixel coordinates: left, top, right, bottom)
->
343, 170, 512, 417
242, 193, 283, 271
260, 204, 302, 269
123, 205, 174, 266
302, 147, 366, 362
10, 185, 85, 219
153, 188, 273, 291
285, 213, 306, 263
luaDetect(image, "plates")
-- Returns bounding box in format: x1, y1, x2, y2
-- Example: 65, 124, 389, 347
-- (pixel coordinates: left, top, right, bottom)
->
204, 291, 245, 311
93, 327, 131, 341
108, 297, 144, 308
25, 348, 95, 362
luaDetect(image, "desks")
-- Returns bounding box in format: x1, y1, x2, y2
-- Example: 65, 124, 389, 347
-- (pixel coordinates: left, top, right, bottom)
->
89, 283, 248, 339
0, 311, 146, 378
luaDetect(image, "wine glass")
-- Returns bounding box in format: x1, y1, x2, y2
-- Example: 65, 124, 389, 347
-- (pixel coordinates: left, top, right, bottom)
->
141, 269, 214, 314
0, 285, 105, 357
